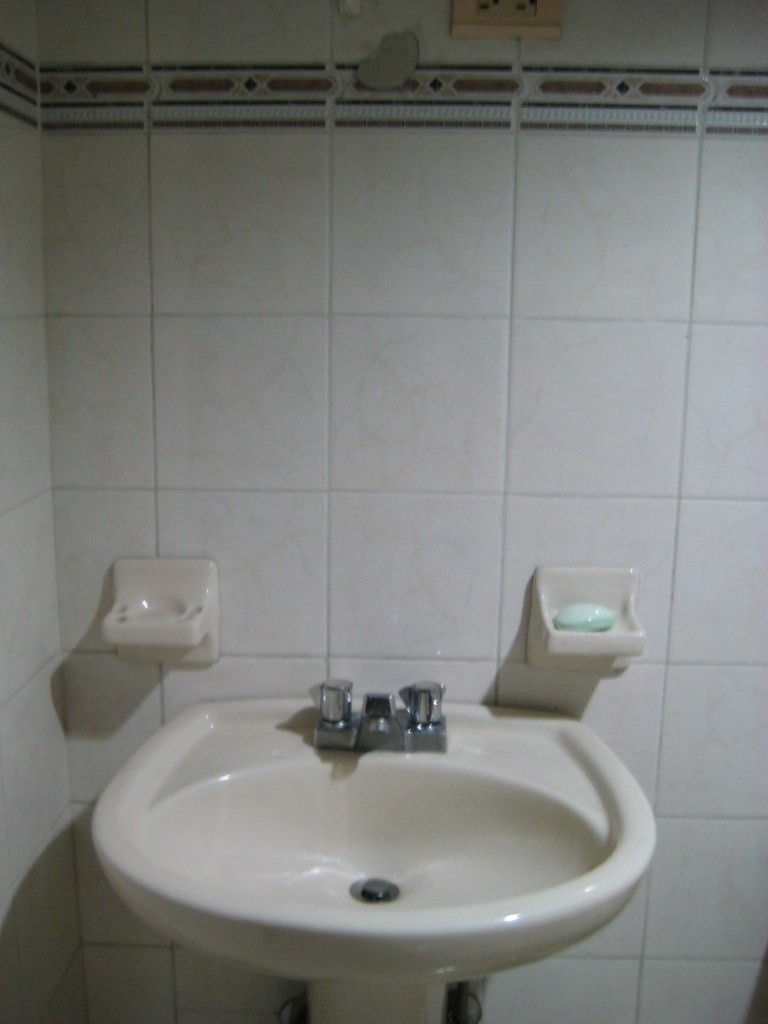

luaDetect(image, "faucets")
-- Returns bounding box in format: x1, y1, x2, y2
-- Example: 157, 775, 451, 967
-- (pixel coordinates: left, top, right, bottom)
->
306, 679, 452, 754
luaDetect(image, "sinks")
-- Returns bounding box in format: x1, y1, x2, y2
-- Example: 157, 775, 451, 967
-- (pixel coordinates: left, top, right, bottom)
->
89, 697, 658, 981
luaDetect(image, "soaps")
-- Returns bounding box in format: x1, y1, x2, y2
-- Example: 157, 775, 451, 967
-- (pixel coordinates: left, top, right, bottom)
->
552, 600, 616, 633
